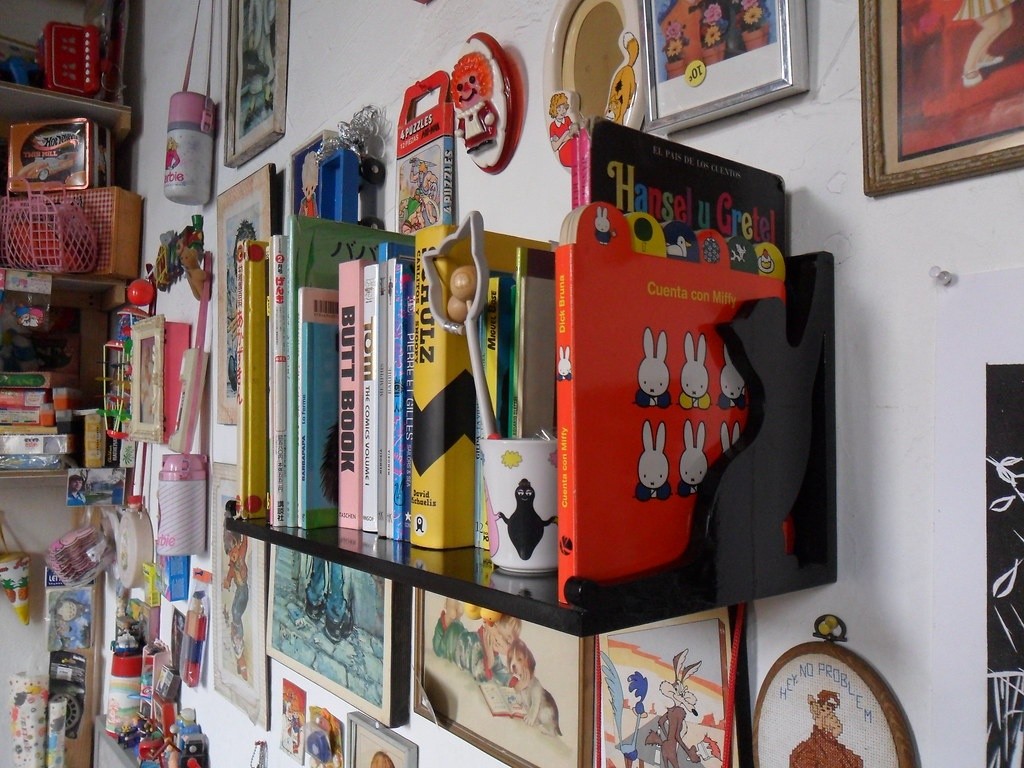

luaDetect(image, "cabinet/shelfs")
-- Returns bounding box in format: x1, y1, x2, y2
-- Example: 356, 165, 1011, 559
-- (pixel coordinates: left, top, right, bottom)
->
0, 79, 132, 483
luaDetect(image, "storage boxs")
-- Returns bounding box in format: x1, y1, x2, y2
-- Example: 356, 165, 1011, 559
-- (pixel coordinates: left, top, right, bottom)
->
8, 118, 116, 193
30, 184, 142, 278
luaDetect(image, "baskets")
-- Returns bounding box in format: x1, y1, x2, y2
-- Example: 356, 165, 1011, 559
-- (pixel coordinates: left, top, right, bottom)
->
0, 177, 97, 273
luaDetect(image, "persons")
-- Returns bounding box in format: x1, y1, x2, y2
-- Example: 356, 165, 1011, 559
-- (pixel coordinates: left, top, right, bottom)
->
67, 475, 123, 506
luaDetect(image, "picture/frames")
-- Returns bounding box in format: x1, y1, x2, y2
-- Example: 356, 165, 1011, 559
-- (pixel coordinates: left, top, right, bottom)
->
266, 541, 414, 729
224, 0, 289, 168
130, 313, 164, 446
210, 462, 271, 731
345, 710, 418, 768
637, 0, 811, 136
858, 0, 1024, 197
288, 129, 338, 224
216, 162, 278, 426
413, 587, 595, 768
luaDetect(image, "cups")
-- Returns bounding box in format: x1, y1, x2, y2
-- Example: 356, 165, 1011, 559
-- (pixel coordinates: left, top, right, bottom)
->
480, 439, 558, 573
155, 454, 214, 558
105, 649, 144, 738
163, 92, 217, 205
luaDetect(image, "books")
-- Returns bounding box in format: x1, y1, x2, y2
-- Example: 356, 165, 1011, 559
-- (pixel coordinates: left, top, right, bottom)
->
236, 115, 787, 608
